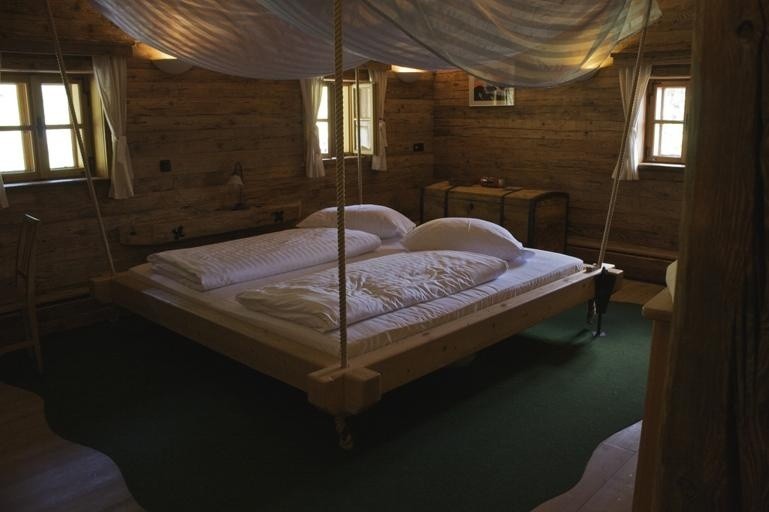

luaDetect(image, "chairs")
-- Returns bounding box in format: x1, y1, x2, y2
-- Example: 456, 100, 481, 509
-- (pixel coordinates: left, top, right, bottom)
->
1, 209, 49, 380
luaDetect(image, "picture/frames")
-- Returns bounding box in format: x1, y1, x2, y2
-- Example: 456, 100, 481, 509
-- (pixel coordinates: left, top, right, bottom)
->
468, 57, 516, 107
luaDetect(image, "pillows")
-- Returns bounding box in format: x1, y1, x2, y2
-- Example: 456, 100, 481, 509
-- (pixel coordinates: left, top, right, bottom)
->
400, 216, 523, 260
296, 199, 418, 246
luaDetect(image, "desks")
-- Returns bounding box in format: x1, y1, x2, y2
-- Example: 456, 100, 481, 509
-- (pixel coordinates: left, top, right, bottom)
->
632, 287, 673, 509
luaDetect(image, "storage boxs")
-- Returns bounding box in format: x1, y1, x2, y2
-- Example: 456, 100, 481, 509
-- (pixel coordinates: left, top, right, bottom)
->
423, 177, 568, 249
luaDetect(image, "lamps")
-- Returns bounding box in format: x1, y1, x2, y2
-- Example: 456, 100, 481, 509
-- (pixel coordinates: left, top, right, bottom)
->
225, 158, 249, 208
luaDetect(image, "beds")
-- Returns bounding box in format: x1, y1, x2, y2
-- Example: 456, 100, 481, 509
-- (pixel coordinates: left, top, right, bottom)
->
35, 0, 657, 444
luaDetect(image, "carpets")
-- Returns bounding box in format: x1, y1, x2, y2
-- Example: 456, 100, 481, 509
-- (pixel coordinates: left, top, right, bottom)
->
2, 295, 671, 511
